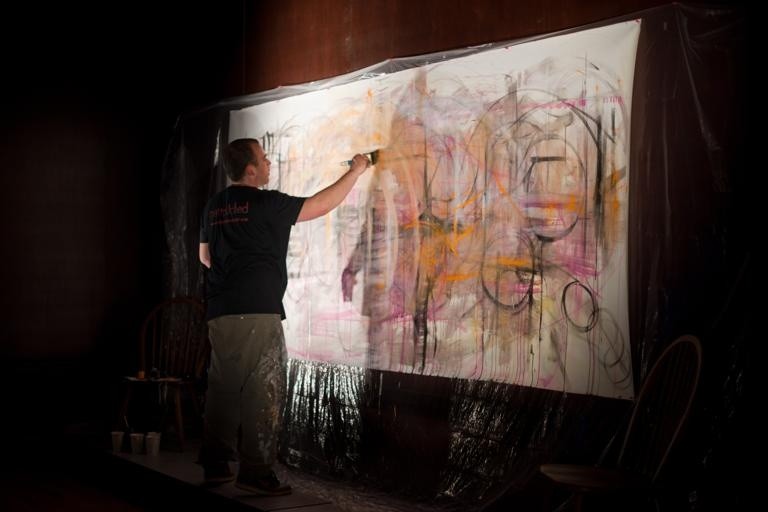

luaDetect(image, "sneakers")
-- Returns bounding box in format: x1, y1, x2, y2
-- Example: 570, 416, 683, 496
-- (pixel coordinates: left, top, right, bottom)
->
234, 465, 291, 495
203, 460, 233, 482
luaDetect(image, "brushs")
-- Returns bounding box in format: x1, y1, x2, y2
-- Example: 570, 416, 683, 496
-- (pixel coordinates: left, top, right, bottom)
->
341, 149, 380, 166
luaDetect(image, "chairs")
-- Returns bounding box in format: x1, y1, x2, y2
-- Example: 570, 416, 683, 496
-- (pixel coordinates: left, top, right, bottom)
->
113, 294, 218, 455
533, 330, 706, 510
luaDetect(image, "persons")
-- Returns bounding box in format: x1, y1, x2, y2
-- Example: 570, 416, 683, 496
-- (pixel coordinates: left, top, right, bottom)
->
200, 137, 370, 496
